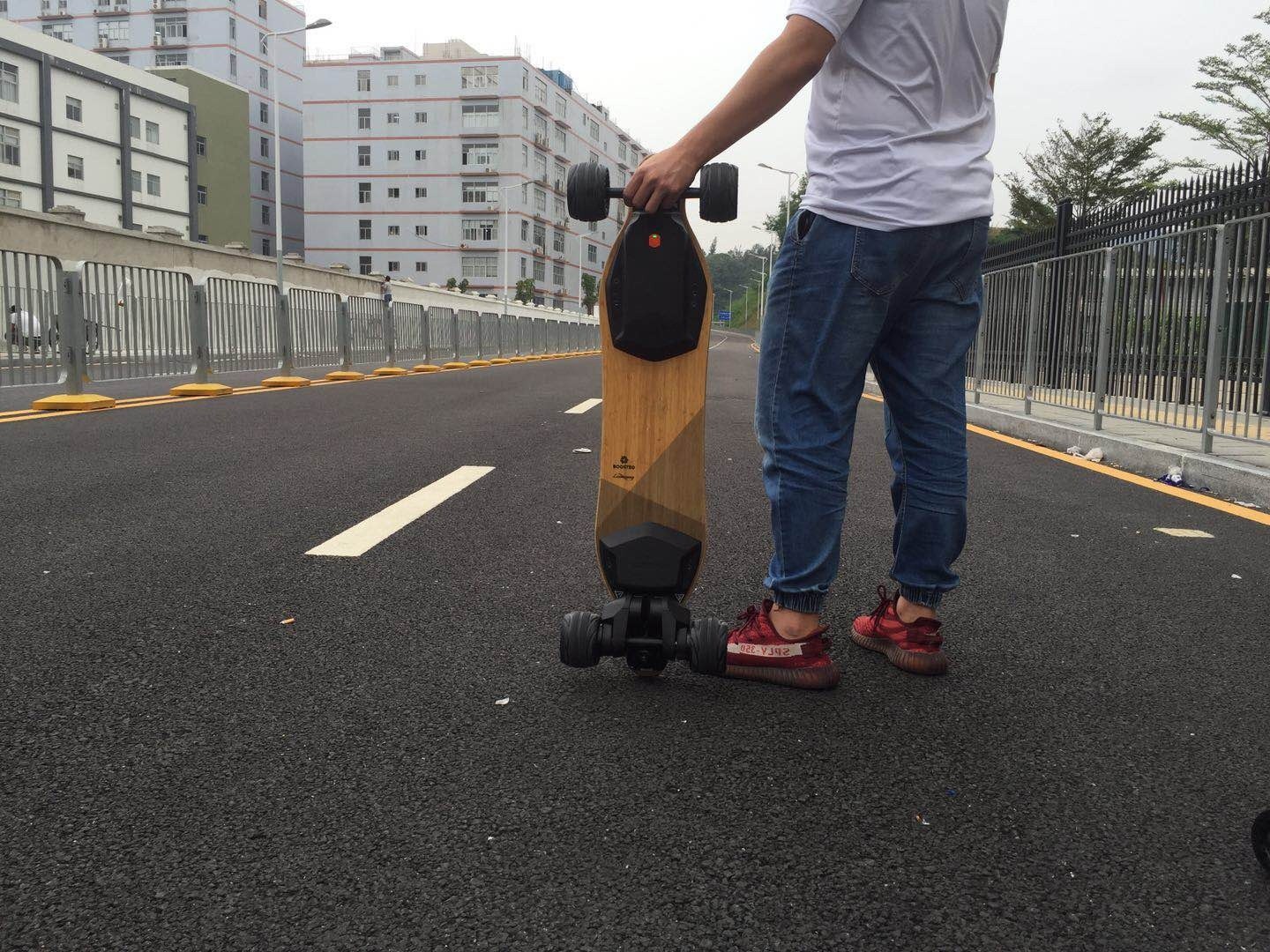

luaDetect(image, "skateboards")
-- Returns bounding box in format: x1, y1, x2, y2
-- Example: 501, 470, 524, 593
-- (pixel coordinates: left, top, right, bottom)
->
561, 161, 740, 685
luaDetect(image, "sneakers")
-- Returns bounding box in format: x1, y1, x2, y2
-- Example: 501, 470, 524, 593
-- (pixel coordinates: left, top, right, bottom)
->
720, 598, 841, 689
852, 583, 944, 674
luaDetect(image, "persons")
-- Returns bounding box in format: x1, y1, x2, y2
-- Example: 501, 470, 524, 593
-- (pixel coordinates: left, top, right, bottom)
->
382, 275, 391, 306
624, 0, 1008, 682
4, 305, 41, 354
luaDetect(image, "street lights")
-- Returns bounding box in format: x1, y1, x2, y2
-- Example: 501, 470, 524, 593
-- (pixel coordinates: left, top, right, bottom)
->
756, 163, 799, 229
751, 225, 778, 278
750, 252, 768, 317
738, 284, 752, 325
723, 288, 733, 329
575, 230, 606, 349
501, 178, 538, 354
749, 269, 767, 332
257, 17, 333, 377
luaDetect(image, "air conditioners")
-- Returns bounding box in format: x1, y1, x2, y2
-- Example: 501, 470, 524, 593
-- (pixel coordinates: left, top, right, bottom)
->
92, 8, 100, 13
458, 242, 467, 249
536, 133, 550, 148
113, 5, 121, 11
153, 0, 162, 9
59, 11, 66, 15
485, 167, 493, 172
99, 37, 107, 48
487, 205, 494, 210
153, 36, 163, 45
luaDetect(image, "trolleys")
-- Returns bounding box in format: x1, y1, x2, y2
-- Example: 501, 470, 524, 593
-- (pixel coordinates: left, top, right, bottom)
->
48, 313, 122, 354
